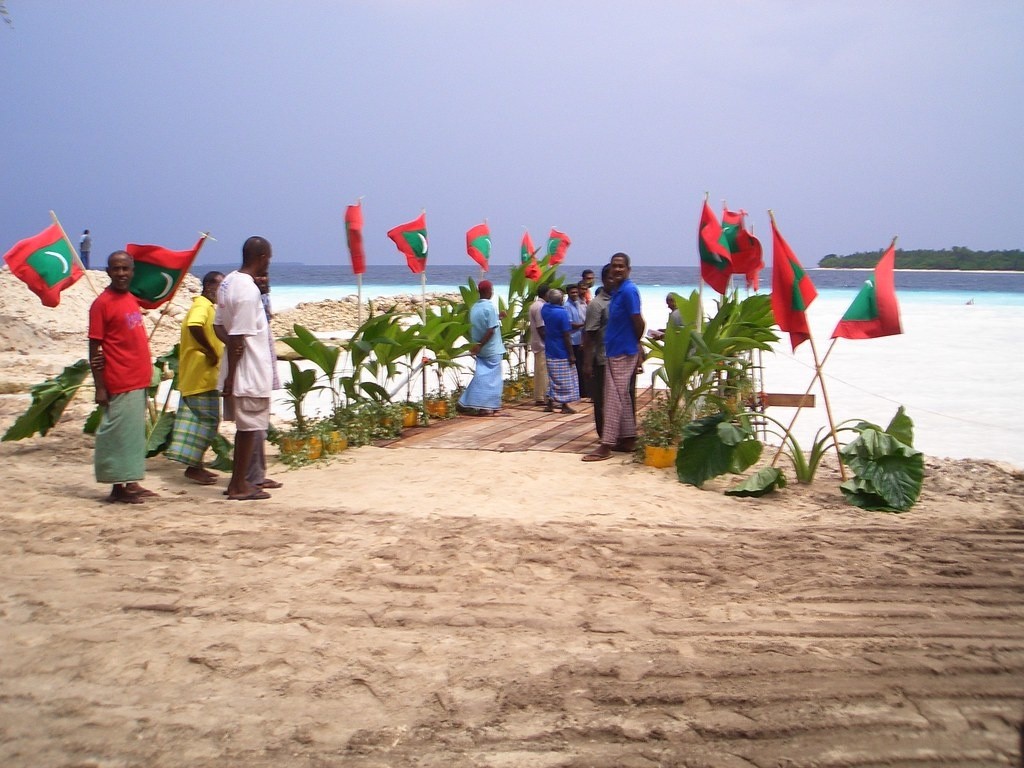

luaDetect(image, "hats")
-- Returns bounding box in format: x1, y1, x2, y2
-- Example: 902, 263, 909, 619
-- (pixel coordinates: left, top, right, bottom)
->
478, 280, 492, 292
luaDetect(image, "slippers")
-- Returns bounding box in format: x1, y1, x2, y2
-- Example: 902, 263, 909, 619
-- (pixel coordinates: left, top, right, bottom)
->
229, 489, 271, 499
255, 478, 281, 488
611, 444, 637, 452
108, 493, 145, 504
581, 452, 613, 461
126, 487, 158, 497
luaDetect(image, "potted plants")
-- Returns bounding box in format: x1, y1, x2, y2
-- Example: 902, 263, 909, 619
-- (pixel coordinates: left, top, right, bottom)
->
265, 246, 569, 472
631, 284, 783, 468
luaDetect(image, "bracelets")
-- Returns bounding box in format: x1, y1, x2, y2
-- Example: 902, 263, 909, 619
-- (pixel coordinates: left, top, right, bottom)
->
477, 342, 482, 346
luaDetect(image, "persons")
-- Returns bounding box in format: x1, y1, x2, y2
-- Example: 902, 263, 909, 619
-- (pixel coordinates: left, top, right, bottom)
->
213, 234, 284, 500
169, 272, 235, 486
80, 229, 91, 270
582, 254, 647, 462
457, 280, 508, 418
528, 269, 595, 414
653, 292, 696, 358
89, 249, 161, 505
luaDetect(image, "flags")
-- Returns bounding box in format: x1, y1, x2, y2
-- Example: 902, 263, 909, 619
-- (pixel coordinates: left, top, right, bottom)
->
387, 214, 429, 274
522, 232, 542, 282
124, 239, 202, 308
3, 222, 84, 307
466, 224, 491, 272
770, 222, 818, 355
345, 205, 368, 275
724, 205, 765, 294
699, 201, 733, 297
832, 247, 903, 341
546, 228, 570, 265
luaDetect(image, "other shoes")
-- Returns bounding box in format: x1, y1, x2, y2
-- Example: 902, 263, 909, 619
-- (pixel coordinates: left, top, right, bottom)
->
493, 411, 511, 416
478, 409, 494, 417
562, 407, 576, 413
545, 408, 553, 412
535, 400, 548, 406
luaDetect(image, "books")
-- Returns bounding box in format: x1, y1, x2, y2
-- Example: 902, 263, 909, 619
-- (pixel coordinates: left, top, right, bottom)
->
647, 328, 665, 340
